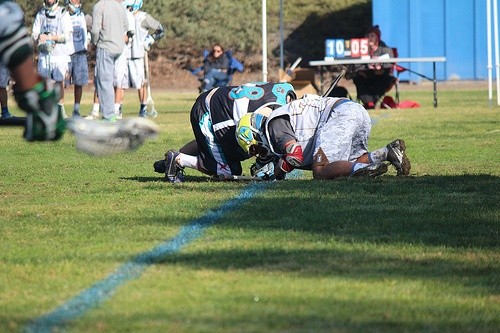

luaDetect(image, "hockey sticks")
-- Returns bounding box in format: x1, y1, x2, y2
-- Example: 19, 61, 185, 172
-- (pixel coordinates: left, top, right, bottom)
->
0, 114, 163, 158
321, 68, 346, 98
218, 173, 267, 183
142, 49, 158, 119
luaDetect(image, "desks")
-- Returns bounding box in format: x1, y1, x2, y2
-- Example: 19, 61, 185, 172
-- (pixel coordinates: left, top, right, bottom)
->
310, 56, 447, 108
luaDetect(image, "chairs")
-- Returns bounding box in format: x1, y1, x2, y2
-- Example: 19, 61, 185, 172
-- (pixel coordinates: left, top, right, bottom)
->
193, 49, 243, 93
357, 48, 399, 107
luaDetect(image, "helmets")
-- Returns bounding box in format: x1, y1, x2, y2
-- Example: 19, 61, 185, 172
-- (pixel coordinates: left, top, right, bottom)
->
235, 112, 271, 156
64, 0, 82, 13
42, 0, 59, 11
121, 0, 143, 14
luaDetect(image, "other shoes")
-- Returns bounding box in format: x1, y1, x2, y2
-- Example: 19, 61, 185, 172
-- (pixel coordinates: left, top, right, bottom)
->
2, 112, 14, 118
101, 117, 117, 122
73, 111, 80, 117
116, 114, 122, 119
85, 114, 99, 120
139, 104, 148, 116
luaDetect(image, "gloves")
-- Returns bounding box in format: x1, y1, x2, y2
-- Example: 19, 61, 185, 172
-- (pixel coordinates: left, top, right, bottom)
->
12, 76, 64, 141
253, 161, 286, 181
250, 155, 280, 176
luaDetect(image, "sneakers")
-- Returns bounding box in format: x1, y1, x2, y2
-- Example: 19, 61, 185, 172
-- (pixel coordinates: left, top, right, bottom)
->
386, 139, 411, 176
154, 160, 165, 173
164, 150, 186, 183
347, 163, 388, 181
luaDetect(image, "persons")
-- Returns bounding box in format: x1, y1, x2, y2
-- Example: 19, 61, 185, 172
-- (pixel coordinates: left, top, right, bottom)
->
84, 0, 165, 124
0, 0, 69, 143
61, 0, 90, 116
234, 94, 412, 182
197, 43, 231, 94
343, 25, 397, 109
32, 0, 75, 119
0, 62, 21, 123
154, 82, 297, 185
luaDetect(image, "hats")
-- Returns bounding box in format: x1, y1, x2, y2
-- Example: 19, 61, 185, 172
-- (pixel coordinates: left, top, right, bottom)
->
364, 26, 381, 50
